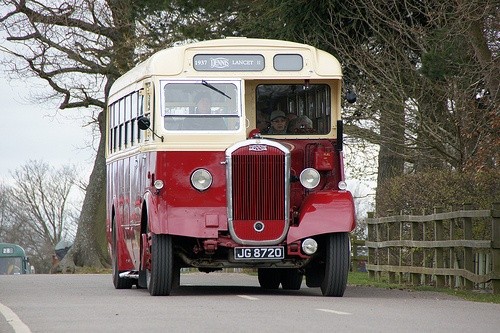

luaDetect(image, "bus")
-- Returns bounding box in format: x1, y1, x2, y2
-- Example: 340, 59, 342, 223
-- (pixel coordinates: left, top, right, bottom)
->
0, 243, 31, 274
106, 37, 357, 297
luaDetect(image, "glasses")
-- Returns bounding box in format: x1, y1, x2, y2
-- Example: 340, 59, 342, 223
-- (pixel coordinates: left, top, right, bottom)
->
272, 119, 285, 122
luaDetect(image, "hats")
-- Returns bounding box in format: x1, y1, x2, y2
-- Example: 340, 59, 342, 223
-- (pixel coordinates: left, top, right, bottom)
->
270, 110, 286, 121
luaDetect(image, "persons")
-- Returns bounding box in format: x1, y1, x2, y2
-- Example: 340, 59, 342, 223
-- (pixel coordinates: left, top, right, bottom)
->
185, 91, 228, 129
291, 115, 313, 134
257, 105, 301, 134
